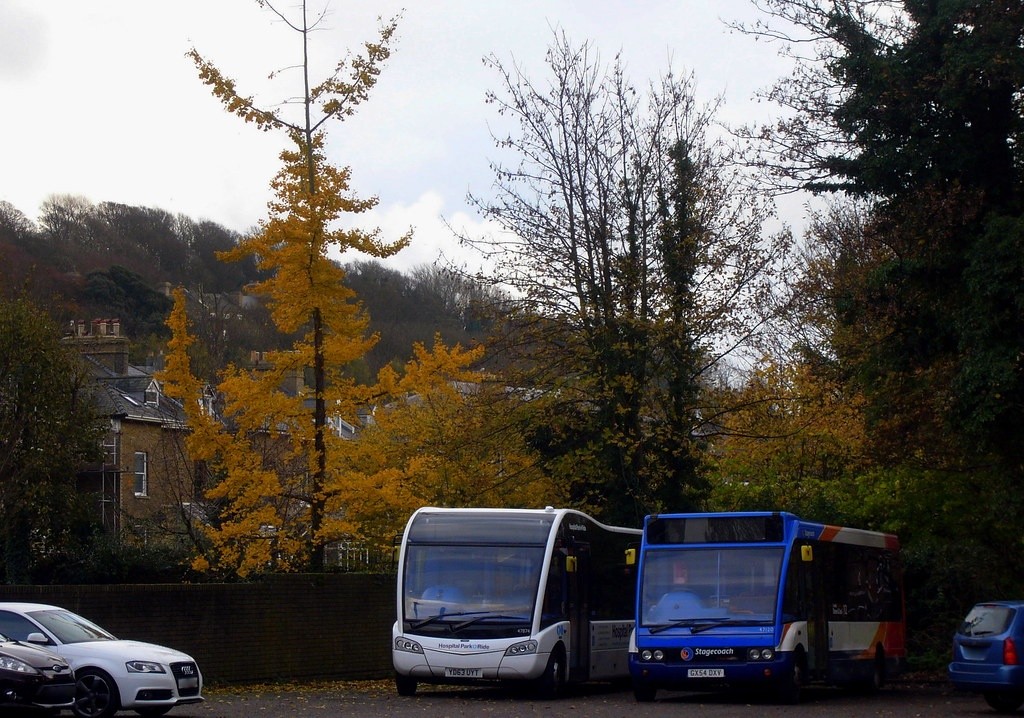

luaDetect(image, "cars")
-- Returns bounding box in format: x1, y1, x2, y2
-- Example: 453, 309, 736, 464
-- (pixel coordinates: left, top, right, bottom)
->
947, 601, 1024, 712
0, 602, 205, 718
0, 630, 78, 718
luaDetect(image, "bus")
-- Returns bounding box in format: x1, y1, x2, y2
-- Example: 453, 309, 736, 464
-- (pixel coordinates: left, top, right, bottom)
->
627, 512, 908, 705
391, 505, 643, 700
